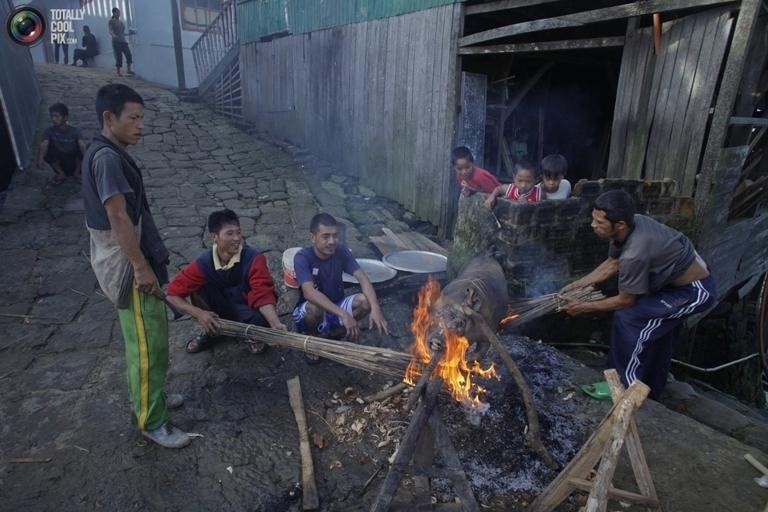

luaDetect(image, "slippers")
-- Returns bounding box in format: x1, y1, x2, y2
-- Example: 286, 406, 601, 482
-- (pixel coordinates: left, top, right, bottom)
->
184, 331, 215, 353
579, 380, 612, 401
50, 175, 65, 186
303, 354, 320, 365
248, 343, 265, 355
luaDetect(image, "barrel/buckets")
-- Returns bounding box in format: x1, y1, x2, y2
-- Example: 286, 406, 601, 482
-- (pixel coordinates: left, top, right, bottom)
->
282, 247, 302, 290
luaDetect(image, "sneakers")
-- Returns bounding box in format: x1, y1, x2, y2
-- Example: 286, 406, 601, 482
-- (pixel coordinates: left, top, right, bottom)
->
166, 393, 185, 410
140, 422, 192, 448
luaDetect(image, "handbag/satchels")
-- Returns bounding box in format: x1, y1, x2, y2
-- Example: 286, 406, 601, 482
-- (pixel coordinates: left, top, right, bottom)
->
83, 216, 143, 310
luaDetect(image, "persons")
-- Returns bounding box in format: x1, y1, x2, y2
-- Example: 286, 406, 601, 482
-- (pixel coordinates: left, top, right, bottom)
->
70, 24, 98, 67
80, 83, 192, 450
36, 102, 88, 186
108, 7, 137, 77
555, 196, 720, 403
53, 10, 70, 66
535, 154, 572, 200
450, 147, 501, 198
483, 159, 547, 213
292, 213, 390, 367
165, 209, 288, 355
